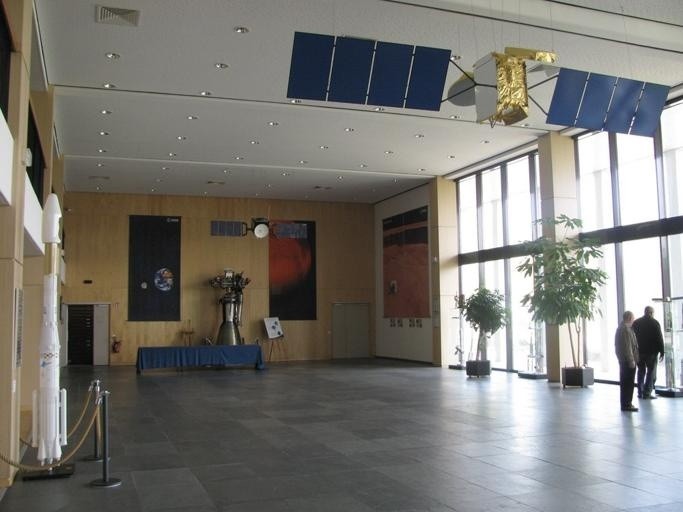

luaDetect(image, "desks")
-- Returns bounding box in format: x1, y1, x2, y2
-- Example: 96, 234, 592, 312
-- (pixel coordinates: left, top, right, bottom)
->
137, 344, 261, 375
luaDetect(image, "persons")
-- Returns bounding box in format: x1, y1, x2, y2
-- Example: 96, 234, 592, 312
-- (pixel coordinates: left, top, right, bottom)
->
614, 310, 640, 411
632, 305, 665, 400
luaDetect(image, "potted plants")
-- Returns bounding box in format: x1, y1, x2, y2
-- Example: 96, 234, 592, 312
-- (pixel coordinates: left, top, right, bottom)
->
514, 213, 611, 390
453, 286, 511, 379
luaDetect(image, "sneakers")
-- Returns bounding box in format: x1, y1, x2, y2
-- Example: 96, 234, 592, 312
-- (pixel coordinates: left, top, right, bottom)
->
621, 405, 639, 412
637, 393, 658, 400
